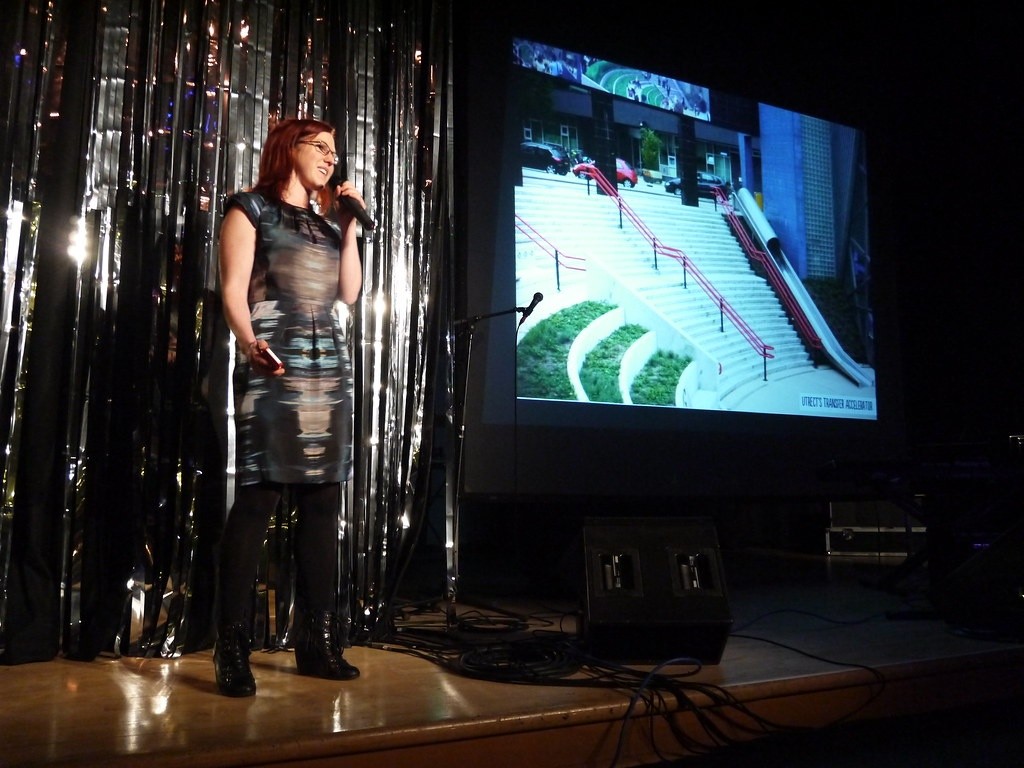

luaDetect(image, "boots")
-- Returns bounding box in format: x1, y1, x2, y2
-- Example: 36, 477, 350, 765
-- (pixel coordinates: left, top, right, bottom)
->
294, 609, 361, 681
212, 617, 257, 698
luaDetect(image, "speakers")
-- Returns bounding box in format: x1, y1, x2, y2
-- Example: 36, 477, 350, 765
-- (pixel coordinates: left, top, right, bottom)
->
578, 517, 733, 666
923, 518, 1024, 639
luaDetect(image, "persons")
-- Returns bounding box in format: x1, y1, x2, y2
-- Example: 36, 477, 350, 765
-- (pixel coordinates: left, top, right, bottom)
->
208, 118, 366, 698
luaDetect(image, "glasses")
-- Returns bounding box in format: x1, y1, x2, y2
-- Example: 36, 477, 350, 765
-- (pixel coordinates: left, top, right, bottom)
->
294, 140, 339, 165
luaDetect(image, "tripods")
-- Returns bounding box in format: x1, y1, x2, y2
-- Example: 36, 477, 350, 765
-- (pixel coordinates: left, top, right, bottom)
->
391, 305, 529, 625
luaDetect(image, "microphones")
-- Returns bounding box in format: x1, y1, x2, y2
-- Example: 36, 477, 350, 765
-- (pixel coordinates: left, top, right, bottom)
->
518, 293, 543, 325
330, 174, 375, 231
